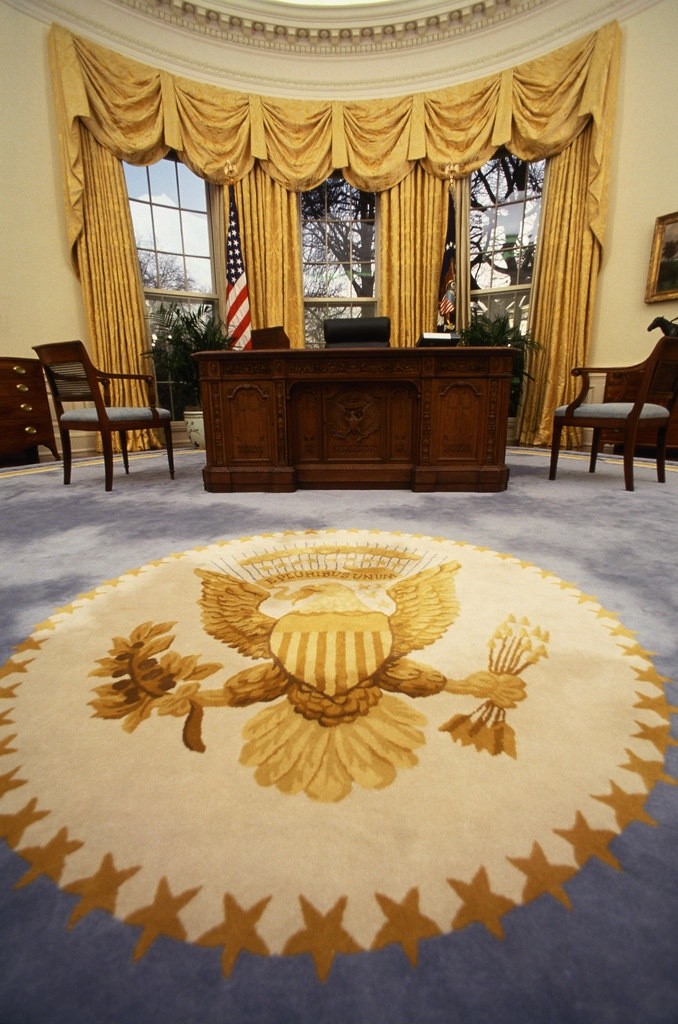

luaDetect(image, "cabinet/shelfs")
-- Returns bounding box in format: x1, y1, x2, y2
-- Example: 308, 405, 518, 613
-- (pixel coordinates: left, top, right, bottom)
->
0, 357, 61, 467
599, 370, 678, 453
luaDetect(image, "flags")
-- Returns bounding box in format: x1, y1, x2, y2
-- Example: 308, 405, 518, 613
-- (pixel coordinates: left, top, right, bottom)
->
225, 185, 252, 352
437, 194, 458, 320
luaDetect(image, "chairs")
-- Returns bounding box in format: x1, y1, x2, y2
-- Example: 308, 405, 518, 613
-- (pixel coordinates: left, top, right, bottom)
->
548, 318, 678, 491
30, 341, 177, 491
322, 316, 392, 350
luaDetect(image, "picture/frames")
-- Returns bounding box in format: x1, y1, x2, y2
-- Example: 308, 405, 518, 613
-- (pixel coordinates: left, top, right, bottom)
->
644, 209, 678, 303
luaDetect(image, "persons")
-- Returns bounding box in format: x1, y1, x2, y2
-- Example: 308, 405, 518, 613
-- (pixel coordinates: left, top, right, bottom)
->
440, 281, 456, 326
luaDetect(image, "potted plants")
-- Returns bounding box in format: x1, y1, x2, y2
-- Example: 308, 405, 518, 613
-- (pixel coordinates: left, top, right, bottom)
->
139, 301, 241, 450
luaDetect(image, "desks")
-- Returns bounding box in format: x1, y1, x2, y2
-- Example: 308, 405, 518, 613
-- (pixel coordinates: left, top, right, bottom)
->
191, 346, 522, 493
